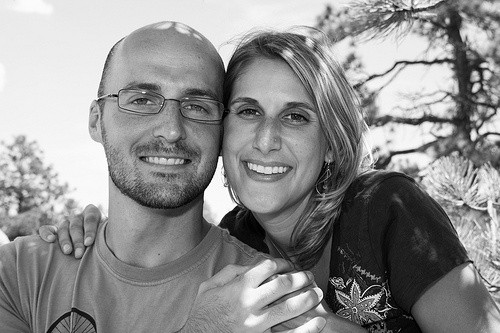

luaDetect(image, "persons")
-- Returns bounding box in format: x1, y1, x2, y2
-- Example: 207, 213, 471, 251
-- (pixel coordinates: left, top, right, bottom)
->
0, 21, 327, 333
38, 26, 500, 333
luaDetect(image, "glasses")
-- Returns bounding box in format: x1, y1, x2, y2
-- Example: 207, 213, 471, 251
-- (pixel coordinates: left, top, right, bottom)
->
97, 89, 226, 121
